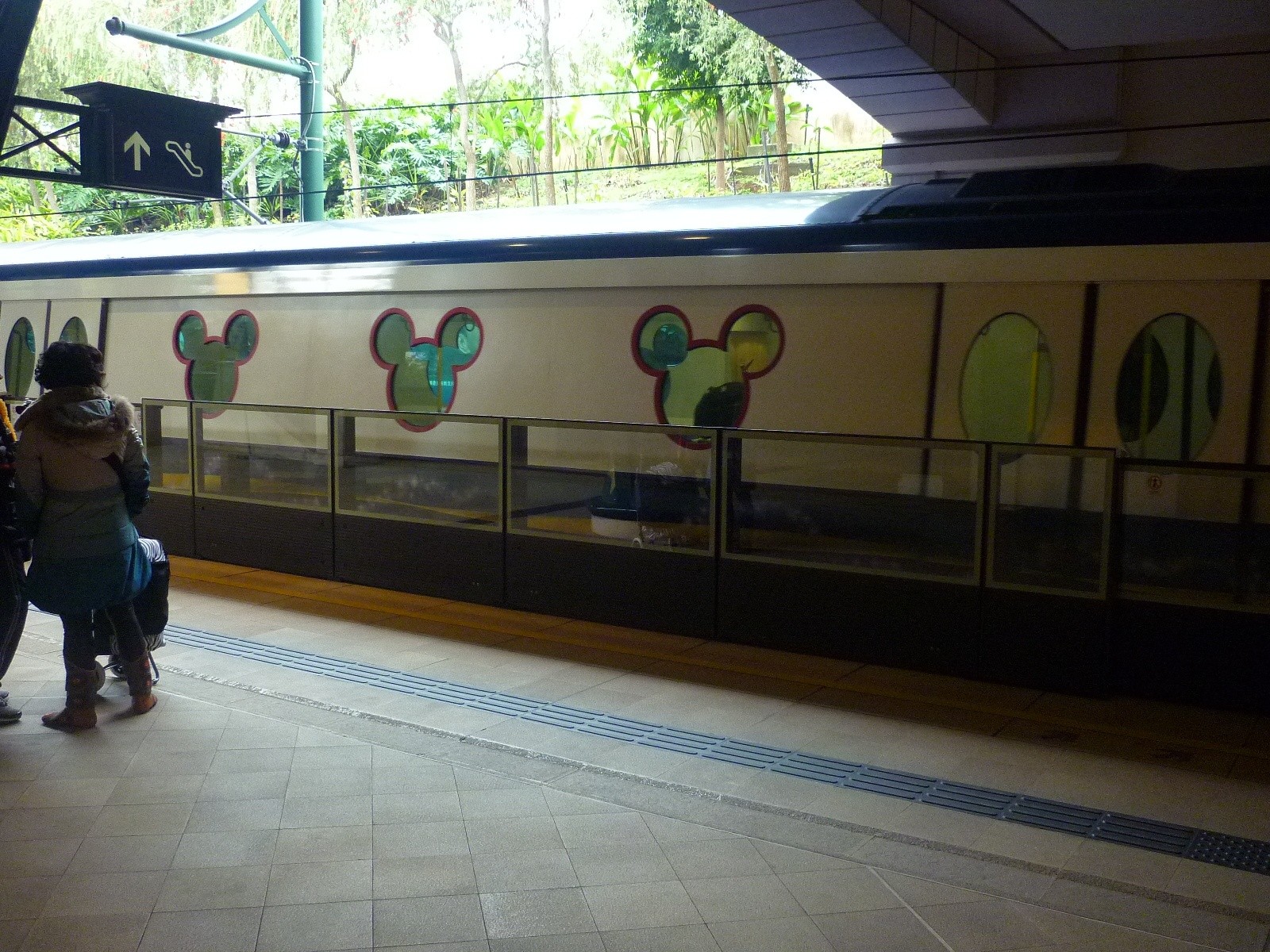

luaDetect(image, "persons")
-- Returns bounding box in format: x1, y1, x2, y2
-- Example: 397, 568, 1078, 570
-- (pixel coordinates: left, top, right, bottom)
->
13, 341, 158, 731
0, 373, 29, 726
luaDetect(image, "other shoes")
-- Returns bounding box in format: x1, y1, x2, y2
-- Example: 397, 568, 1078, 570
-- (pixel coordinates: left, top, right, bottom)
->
0, 690, 22, 721
42, 707, 98, 728
131, 693, 158, 711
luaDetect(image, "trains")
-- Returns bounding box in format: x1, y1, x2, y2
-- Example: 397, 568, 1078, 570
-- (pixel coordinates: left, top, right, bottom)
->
0, 164, 1270, 619
